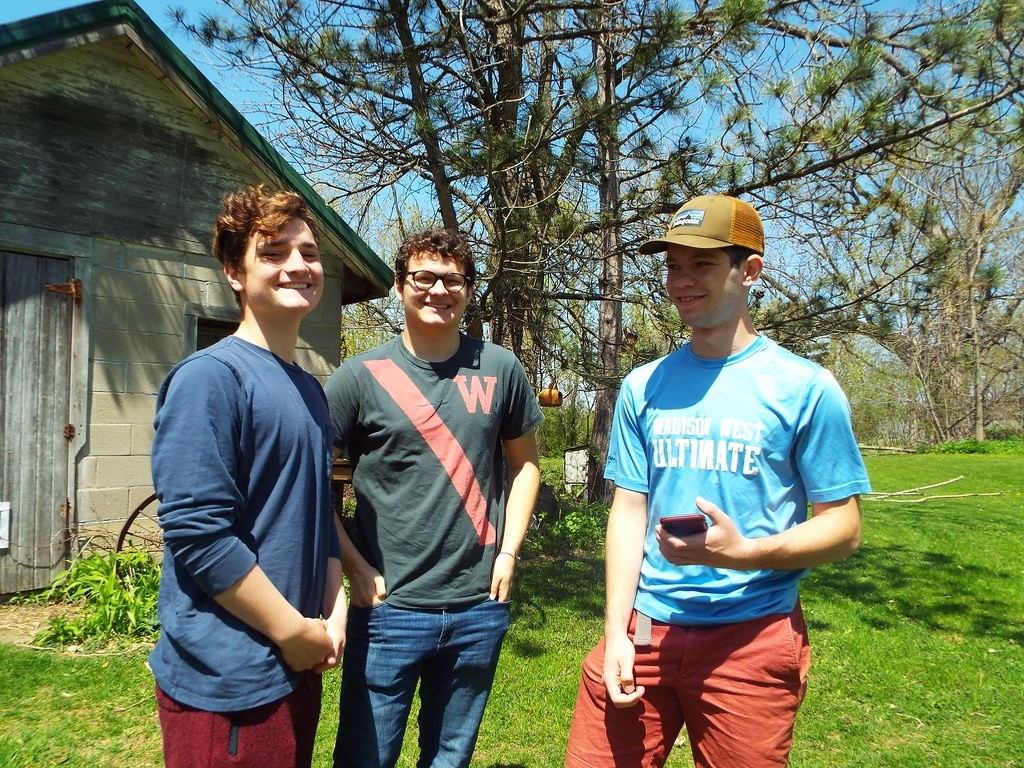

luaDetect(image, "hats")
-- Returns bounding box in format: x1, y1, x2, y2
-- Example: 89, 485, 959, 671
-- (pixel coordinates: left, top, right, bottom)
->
638, 192, 765, 257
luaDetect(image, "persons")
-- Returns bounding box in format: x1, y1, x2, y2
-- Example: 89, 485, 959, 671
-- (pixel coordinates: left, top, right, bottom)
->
321, 232, 541, 768
565, 196, 876, 767
150, 183, 350, 768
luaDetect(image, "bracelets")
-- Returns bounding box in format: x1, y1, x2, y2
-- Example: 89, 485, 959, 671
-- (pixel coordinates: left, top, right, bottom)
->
499, 552, 521, 565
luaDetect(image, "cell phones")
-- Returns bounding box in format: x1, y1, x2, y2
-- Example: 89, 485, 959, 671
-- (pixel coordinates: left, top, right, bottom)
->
660, 515, 708, 538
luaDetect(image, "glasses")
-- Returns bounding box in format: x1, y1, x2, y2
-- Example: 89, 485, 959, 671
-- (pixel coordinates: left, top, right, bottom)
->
401, 271, 472, 293
